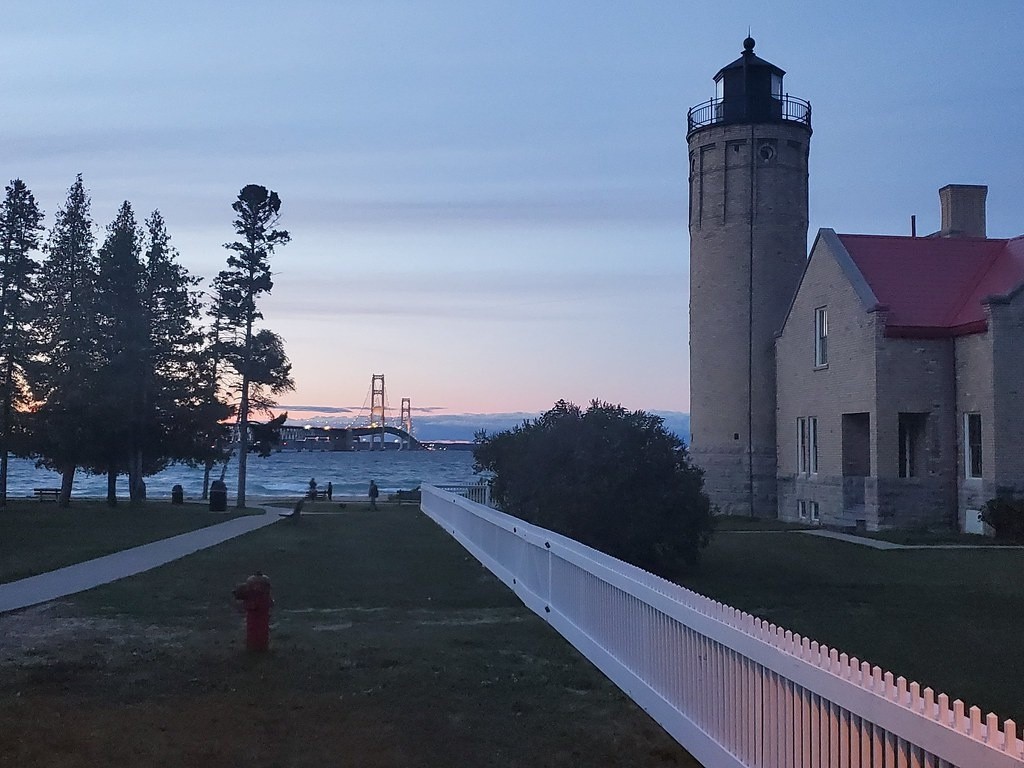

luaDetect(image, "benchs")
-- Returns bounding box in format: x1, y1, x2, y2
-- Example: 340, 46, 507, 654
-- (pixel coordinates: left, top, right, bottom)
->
397, 489, 421, 507
305, 490, 327, 502
33, 487, 62, 502
278, 499, 303, 522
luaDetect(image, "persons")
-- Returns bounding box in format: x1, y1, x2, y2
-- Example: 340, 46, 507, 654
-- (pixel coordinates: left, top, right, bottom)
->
309, 478, 318, 503
368, 480, 379, 511
326, 482, 333, 500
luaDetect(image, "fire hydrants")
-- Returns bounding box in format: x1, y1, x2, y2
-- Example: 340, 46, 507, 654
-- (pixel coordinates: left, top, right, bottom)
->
231, 569, 271, 646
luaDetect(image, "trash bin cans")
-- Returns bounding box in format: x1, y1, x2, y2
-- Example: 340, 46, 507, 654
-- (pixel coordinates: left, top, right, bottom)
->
210, 481, 228, 510
172, 485, 183, 504
